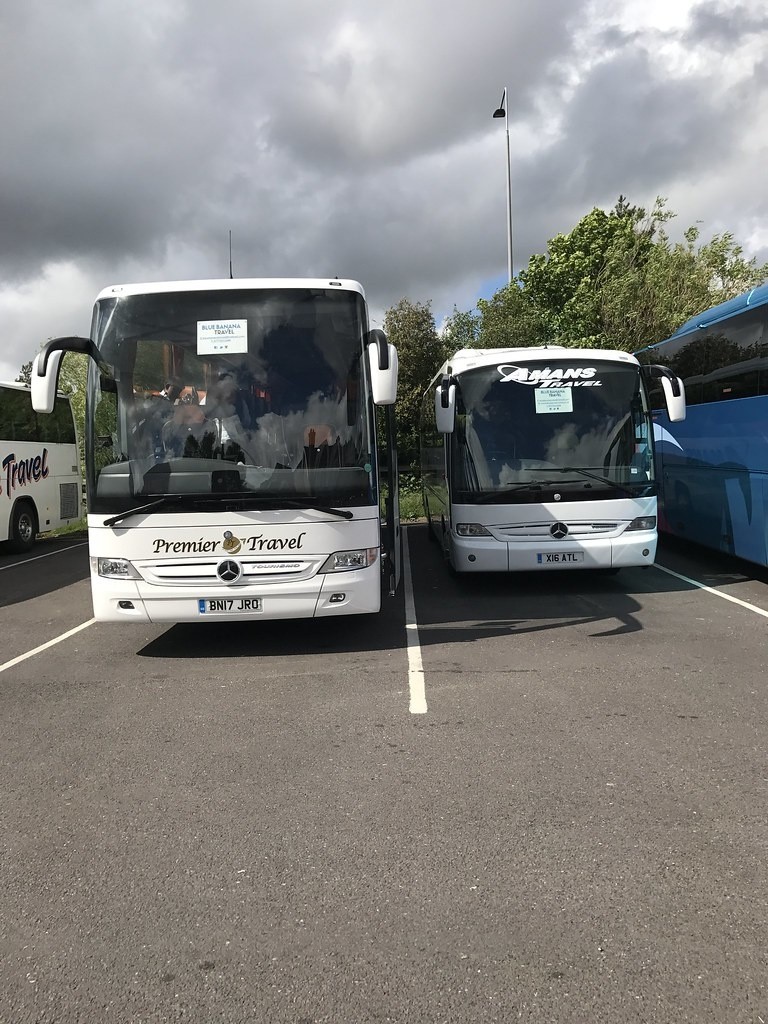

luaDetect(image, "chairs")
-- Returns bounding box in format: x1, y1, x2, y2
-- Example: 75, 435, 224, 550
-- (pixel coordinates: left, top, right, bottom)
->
161, 418, 219, 457
300, 424, 338, 447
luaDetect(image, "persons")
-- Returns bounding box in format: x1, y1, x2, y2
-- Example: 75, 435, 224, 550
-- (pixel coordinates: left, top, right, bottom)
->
158, 376, 184, 405
198, 373, 253, 428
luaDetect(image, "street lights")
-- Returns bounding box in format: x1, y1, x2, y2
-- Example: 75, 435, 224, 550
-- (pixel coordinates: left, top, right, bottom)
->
492, 86, 513, 283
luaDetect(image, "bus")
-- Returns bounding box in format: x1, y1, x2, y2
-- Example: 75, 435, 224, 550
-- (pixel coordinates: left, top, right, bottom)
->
30, 278, 399, 624
622, 287, 768, 568
417, 344, 687, 587
0, 381, 82, 551
